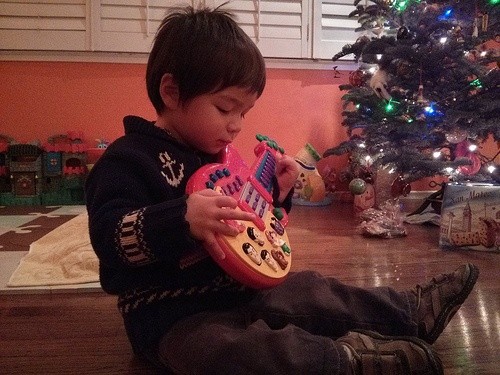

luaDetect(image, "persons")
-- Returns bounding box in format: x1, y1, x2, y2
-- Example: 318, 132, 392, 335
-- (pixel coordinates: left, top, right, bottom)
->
83, 9, 479, 375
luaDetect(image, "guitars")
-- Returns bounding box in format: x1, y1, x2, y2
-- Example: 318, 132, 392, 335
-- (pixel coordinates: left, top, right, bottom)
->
185, 136, 297, 289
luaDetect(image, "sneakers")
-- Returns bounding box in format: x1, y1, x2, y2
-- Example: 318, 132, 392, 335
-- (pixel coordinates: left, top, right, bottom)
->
417, 262, 480, 346
340, 326, 443, 375
